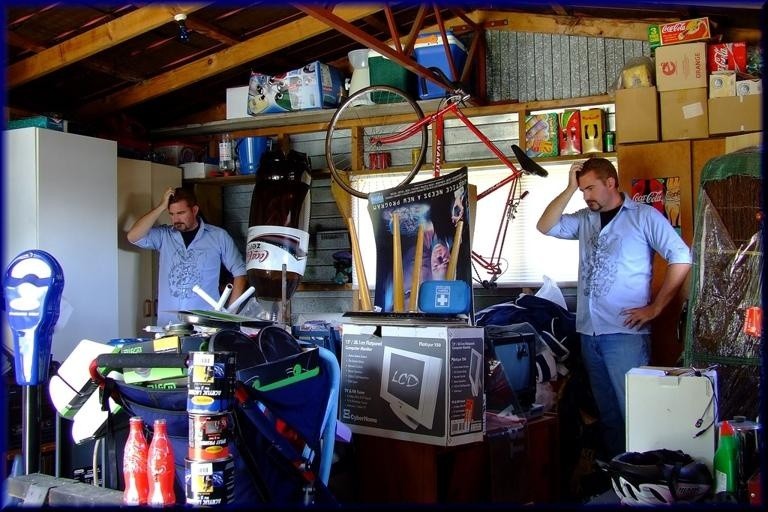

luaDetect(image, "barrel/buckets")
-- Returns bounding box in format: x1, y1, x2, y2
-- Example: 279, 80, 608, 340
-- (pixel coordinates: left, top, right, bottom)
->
234, 135, 270, 174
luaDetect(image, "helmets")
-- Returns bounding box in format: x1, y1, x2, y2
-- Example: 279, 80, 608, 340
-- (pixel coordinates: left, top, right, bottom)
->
609, 447, 713, 506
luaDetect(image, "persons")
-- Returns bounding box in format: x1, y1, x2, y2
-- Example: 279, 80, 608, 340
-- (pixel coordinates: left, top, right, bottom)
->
127, 186, 246, 328
420, 187, 465, 281
537, 157, 693, 435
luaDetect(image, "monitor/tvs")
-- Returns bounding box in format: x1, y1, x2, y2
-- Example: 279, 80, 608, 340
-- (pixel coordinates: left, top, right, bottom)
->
468, 348, 483, 397
489, 331, 537, 412
379, 346, 443, 431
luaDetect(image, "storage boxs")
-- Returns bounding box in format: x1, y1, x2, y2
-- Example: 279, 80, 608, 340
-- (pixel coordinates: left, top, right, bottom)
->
655, 42, 709, 91
367, 45, 415, 104
707, 94, 763, 137
661, 88, 707, 142
613, 86, 660, 145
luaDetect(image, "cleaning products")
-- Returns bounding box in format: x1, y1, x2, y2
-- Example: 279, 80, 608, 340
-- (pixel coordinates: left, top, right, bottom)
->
714, 420, 741, 495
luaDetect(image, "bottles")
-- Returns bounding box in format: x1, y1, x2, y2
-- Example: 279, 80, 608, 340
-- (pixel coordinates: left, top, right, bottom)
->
121, 417, 150, 505
243, 148, 314, 327
148, 418, 177, 508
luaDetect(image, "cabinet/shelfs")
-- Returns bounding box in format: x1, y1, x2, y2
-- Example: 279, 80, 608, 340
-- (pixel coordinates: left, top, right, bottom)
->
116, 157, 184, 339
616, 134, 762, 366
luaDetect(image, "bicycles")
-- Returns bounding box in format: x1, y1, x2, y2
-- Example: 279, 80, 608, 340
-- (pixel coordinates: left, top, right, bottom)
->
324, 65, 549, 290
